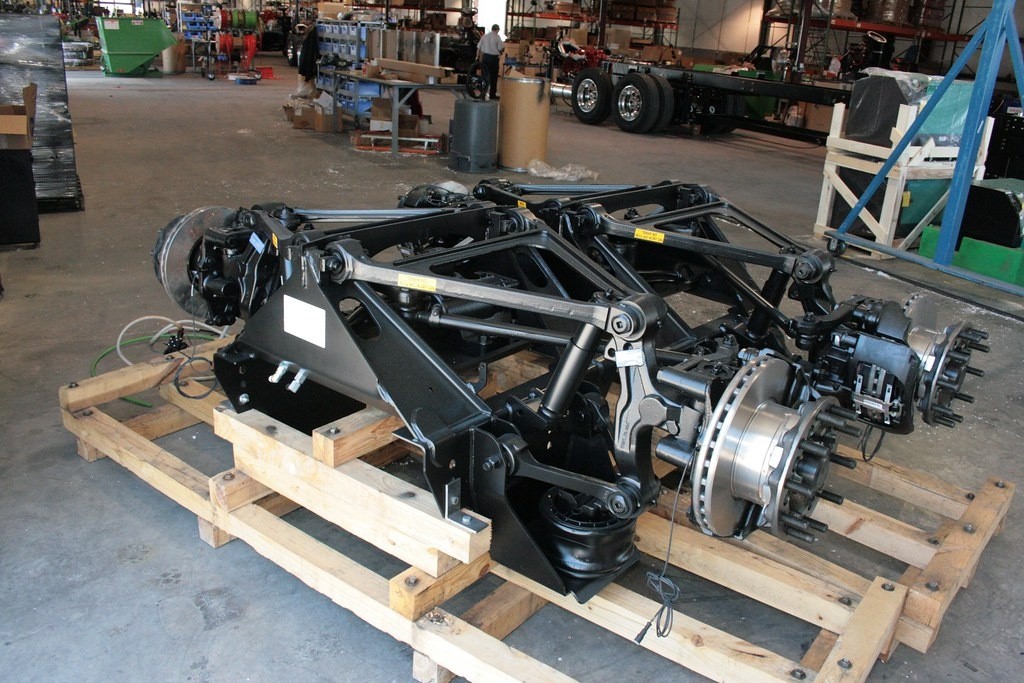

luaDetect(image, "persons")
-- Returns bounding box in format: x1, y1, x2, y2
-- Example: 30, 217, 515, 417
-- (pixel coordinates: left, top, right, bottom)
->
475, 25, 505, 100
308, 8, 318, 20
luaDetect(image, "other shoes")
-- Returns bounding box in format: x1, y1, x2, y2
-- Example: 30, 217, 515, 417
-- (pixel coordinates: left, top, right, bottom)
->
489, 93, 500, 100
481, 93, 486, 100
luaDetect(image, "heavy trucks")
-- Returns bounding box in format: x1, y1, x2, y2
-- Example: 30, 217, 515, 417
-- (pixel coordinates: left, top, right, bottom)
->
571, 57, 1024, 180
